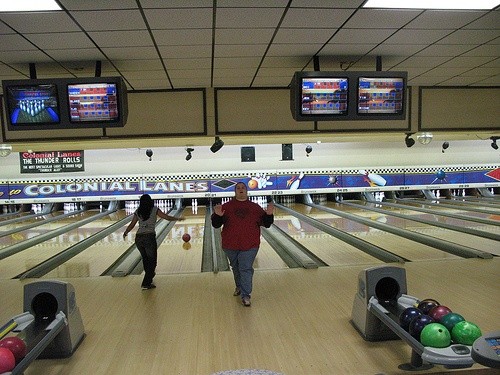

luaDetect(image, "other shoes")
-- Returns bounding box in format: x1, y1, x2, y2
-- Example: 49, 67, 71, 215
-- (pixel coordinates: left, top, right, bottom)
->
241, 295, 251, 305
141, 284, 156, 290
233, 287, 242, 296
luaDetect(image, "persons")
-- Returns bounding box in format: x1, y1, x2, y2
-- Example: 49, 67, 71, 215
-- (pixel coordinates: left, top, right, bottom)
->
123, 193, 186, 290
209, 182, 274, 307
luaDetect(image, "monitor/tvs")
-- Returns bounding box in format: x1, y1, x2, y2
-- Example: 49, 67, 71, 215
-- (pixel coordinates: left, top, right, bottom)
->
3, 76, 128, 131
290, 70, 408, 121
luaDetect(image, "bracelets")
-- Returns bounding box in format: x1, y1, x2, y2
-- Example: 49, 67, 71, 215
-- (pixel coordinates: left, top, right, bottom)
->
124, 230, 129, 235
177, 217, 179, 220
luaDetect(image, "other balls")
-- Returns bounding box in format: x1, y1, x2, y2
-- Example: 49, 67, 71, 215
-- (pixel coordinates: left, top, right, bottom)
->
182, 233, 191, 242
400, 299, 482, 348
0, 337, 27, 374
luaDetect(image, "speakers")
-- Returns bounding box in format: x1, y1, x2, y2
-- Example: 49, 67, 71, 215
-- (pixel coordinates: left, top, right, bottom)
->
282, 144, 292, 160
241, 147, 255, 161
